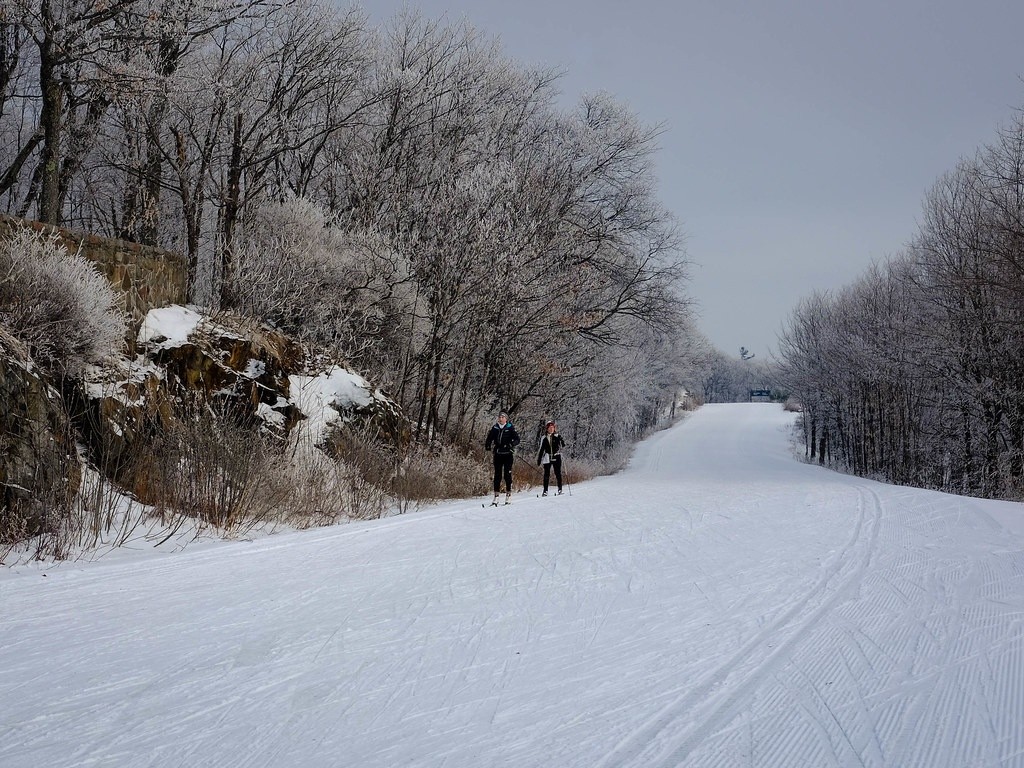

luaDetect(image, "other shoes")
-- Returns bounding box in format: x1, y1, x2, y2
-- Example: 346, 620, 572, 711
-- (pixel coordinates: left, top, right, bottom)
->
558, 489, 562, 494
542, 490, 548, 496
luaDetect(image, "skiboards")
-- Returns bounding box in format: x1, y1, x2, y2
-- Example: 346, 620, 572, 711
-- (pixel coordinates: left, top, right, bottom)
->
536, 493, 565, 499
481, 503, 512, 508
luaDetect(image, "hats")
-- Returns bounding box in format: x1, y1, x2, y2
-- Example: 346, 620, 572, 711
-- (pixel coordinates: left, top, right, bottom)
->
499, 409, 509, 419
545, 421, 554, 429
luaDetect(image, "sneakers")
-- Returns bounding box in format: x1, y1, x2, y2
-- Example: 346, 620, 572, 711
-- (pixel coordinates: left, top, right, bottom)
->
505, 494, 512, 505
492, 497, 499, 507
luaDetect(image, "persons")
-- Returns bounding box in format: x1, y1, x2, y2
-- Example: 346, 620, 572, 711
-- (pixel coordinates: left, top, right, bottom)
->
484, 409, 520, 507
537, 420, 566, 496
